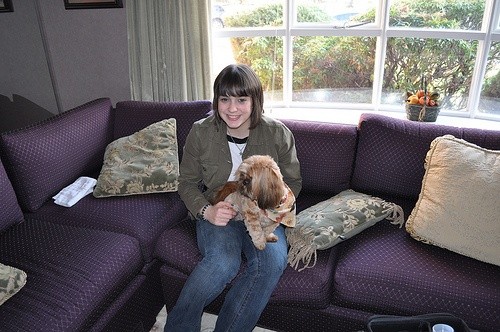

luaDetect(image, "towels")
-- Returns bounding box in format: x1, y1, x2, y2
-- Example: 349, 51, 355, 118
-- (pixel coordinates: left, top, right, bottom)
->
53, 176, 97, 208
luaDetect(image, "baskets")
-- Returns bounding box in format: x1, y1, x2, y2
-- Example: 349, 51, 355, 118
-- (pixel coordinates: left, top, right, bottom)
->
406, 74, 441, 122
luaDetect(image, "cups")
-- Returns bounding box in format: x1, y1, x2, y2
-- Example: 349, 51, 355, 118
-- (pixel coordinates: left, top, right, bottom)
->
433, 324, 454, 332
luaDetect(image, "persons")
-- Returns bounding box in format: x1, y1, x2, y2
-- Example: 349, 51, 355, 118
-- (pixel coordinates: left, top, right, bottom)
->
164, 64, 302, 332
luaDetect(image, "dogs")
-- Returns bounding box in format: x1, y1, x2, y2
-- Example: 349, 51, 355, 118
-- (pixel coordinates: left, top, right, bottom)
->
185, 152, 298, 251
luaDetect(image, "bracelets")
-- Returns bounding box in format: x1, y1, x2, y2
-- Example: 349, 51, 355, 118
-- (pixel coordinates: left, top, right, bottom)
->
202, 204, 211, 220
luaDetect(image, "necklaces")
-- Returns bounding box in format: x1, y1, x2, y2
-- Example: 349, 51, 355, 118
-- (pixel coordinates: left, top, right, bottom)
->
226, 127, 246, 155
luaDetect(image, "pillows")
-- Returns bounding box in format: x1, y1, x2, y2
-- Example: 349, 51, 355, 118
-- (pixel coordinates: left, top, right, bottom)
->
0, 262, 28, 306
406, 133, 500, 265
283, 188, 406, 272
93, 117, 179, 198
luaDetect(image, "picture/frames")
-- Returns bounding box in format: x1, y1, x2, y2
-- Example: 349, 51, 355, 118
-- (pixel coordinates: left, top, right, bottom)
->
64, 0, 123, 9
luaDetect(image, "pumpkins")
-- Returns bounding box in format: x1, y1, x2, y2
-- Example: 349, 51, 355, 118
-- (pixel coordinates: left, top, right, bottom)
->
404, 89, 440, 106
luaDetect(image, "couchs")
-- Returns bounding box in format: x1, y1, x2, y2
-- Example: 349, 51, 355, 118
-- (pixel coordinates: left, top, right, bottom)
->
0, 97, 500, 332
